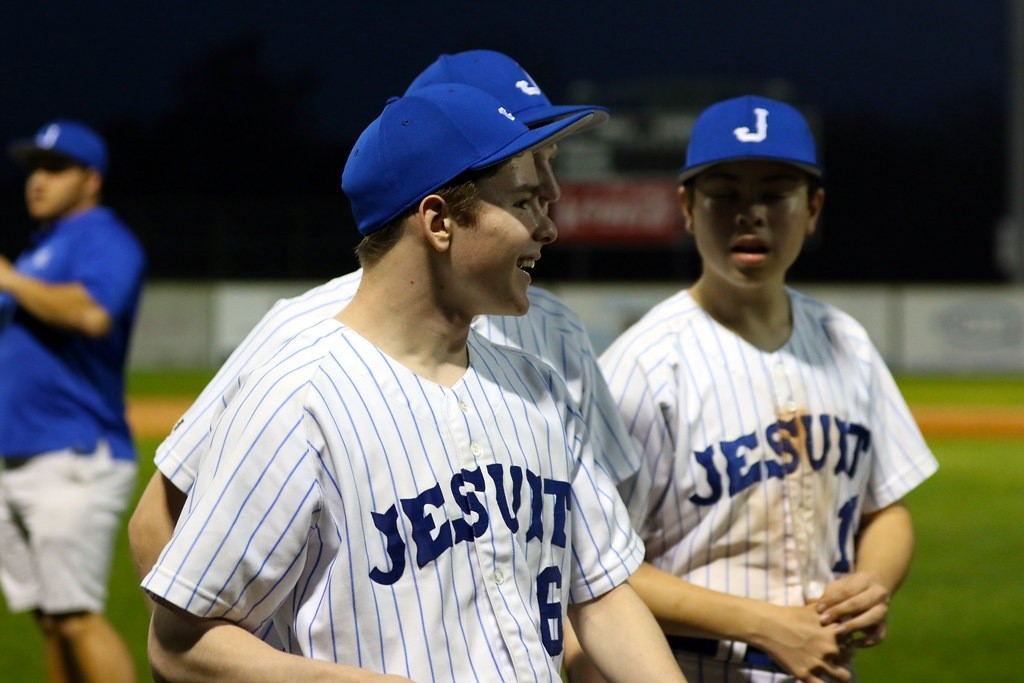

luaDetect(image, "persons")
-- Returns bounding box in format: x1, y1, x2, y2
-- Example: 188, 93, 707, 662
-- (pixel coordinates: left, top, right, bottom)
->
129, 52, 642, 683
598, 99, 937, 683
0, 124, 140, 683
141, 87, 693, 683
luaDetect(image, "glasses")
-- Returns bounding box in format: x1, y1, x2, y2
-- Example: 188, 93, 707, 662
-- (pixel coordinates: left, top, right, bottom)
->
17, 154, 88, 174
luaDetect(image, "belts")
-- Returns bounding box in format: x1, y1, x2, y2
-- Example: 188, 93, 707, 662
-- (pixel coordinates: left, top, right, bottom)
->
665, 634, 785, 672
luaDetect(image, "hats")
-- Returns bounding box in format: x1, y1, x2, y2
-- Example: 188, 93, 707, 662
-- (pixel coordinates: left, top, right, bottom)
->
10, 121, 108, 174
341, 83, 596, 237
403, 48, 611, 136
676, 94, 822, 185
